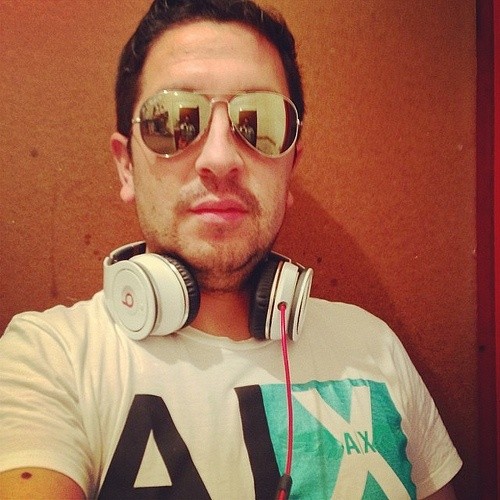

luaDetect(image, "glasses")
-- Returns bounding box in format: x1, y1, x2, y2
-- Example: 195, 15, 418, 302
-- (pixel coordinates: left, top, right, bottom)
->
128, 88, 301, 159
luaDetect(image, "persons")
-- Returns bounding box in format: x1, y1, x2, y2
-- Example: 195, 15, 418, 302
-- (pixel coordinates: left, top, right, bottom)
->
240, 118, 255, 144
1, 0, 464, 500
178, 115, 195, 144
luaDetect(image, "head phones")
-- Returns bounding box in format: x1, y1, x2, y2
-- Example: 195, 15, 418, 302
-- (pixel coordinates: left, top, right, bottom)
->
103, 241, 313, 343
